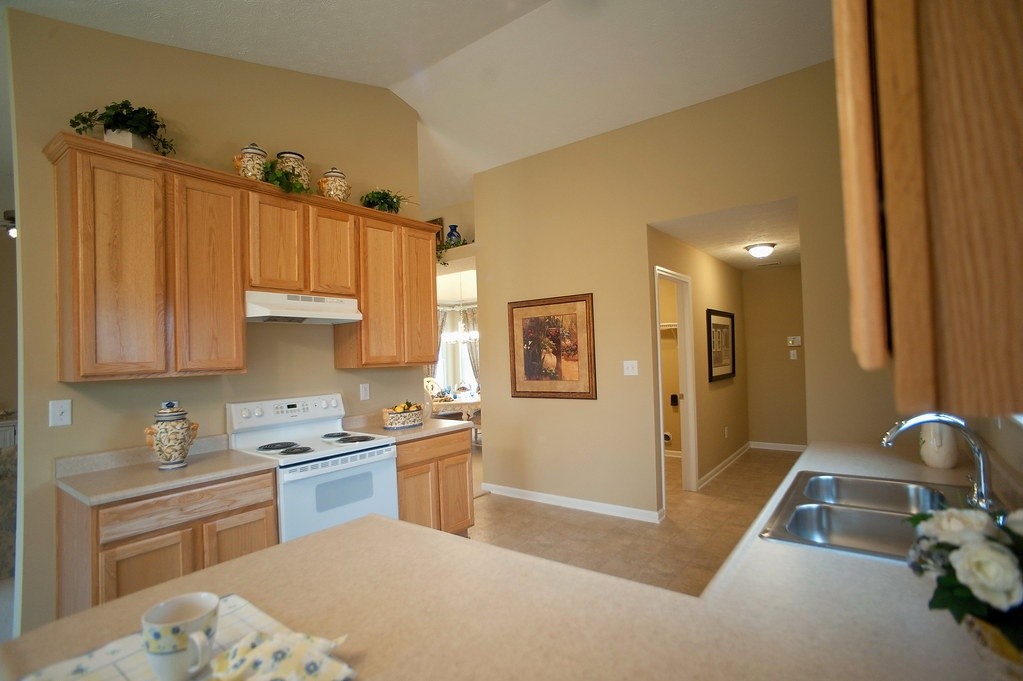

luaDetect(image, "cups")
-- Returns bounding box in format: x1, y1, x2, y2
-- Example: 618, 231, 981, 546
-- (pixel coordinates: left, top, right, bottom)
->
141, 592, 219, 681
918, 422, 959, 470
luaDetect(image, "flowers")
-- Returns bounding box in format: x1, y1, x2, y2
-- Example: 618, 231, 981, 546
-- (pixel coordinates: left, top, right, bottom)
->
902, 504, 1023, 652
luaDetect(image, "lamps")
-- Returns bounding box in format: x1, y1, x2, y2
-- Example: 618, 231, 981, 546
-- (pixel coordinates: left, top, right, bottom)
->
744, 243, 777, 259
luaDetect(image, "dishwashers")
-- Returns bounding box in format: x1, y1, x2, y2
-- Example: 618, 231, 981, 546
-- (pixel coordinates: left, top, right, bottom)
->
276, 445, 399, 543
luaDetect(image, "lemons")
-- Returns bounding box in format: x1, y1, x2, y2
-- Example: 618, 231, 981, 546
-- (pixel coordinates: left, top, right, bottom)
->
395, 403, 418, 412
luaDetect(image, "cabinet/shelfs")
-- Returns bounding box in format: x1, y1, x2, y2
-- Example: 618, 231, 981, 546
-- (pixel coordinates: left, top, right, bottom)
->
396, 427, 475, 537
55, 449, 280, 619
830, 0, 1023, 425
40, 128, 442, 385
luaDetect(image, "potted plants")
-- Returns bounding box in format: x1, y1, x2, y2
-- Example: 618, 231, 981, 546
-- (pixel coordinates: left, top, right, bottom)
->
359, 186, 420, 215
69, 100, 176, 157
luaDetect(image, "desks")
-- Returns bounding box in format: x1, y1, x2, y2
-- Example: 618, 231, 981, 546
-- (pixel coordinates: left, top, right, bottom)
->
433, 390, 480, 421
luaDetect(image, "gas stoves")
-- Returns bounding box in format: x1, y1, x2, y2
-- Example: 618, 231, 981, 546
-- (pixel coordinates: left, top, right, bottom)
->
226, 393, 396, 466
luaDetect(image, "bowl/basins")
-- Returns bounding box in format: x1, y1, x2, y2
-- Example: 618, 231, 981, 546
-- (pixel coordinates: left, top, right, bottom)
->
381, 411, 423, 430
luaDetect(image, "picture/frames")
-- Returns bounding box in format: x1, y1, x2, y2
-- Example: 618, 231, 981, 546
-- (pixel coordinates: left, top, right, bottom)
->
706, 308, 736, 382
507, 293, 598, 400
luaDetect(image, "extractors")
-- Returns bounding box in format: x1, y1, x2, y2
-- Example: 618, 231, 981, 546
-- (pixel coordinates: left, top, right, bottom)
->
244, 291, 363, 324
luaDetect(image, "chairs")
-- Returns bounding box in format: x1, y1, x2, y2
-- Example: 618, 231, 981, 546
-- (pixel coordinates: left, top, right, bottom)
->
424, 376, 482, 446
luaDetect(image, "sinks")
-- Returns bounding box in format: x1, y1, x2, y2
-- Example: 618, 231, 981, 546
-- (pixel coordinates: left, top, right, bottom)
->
758, 501, 918, 559
791, 469, 951, 517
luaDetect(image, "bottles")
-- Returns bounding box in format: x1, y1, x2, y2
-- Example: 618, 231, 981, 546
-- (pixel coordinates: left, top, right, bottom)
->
233, 143, 268, 181
317, 167, 350, 202
275, 151, 311, 190
144, 400, 199, 471
447, 225, 461, 242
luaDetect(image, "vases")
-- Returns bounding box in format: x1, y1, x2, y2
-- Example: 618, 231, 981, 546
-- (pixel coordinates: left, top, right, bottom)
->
963, 613, 1023, 681
447, 225, 461, 245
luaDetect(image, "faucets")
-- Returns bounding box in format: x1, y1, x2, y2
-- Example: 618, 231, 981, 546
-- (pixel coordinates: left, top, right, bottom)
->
881, 411, 991, 512
